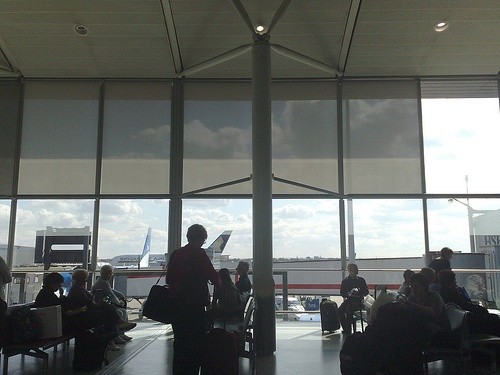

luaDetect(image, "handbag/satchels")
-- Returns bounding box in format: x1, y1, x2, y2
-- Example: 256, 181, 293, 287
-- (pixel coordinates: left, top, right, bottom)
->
431, 328, 460, 349
142, 284, 176, 324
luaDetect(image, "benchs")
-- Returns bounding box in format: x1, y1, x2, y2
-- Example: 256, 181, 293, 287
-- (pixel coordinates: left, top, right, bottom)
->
0, 300, 111, 375
354, 284, 500, 375
212, 298, 257, 375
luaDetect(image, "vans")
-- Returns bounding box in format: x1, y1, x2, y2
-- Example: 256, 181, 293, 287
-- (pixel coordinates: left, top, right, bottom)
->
274, 294, 308, 321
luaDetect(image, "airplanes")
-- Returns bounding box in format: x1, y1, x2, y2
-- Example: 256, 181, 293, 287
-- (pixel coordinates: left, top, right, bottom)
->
109, 226, 233, 269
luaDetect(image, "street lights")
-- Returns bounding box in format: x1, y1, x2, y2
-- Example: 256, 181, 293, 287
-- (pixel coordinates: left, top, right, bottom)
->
448, 196, 475, 254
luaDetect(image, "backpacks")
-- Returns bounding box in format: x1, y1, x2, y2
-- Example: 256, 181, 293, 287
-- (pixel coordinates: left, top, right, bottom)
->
9, 306, 35, 345
339, 333, 378, 375
72, 328, 105, 371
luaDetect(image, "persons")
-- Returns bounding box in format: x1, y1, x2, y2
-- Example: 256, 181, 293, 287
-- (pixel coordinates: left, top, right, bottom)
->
337, 263, 369, 342
35, 265, 136, 370
165, 224, 219, 375
437, 270, 500, 375
206, 260, 252, 312
398, 270, 417, 300
339, 301, 431, 375
429, 248, 454, 274
396, 273, 452, 349
205, 269, 241, 333
396, 267, 436, 302
0, 256, 12, 304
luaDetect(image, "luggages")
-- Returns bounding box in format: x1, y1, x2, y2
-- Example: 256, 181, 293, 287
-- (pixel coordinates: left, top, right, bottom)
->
201, 328, 240, 375
320, 299, 340, 335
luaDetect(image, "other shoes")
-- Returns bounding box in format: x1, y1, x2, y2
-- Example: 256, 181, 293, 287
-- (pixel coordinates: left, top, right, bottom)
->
119, 334, 132, 340
113, 335, 126, 343
116, 321, 136, 332
108, 343, 121, 351
